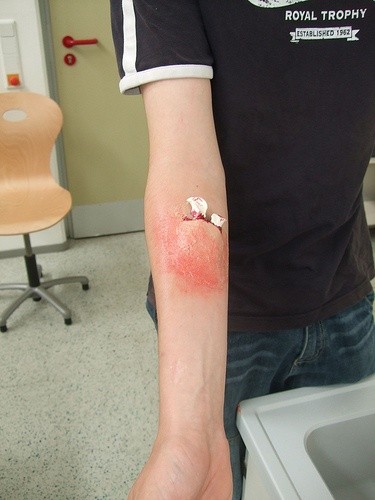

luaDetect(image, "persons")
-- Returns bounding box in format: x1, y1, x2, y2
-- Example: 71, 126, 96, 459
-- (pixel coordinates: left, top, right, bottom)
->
108, 0, 375, 500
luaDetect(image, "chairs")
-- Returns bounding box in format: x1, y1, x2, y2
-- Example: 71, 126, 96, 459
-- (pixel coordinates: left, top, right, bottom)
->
0, 88, 90, 332
